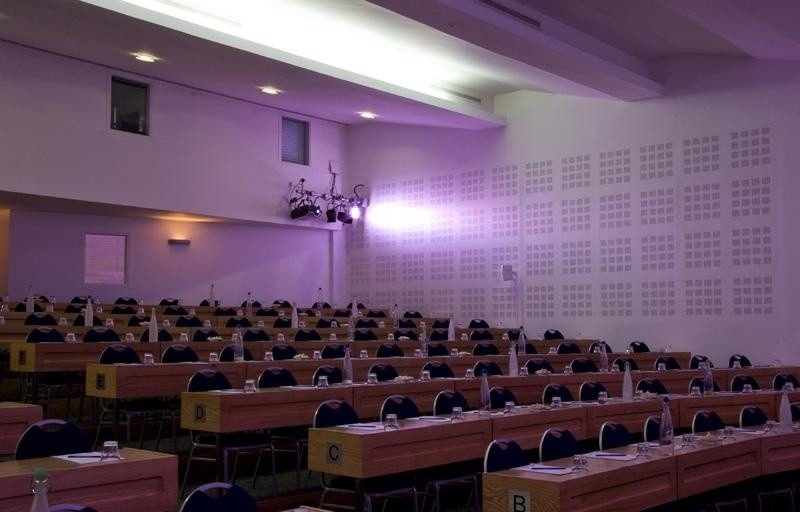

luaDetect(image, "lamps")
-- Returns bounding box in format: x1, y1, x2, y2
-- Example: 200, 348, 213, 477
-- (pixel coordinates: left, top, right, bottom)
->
326, 208, 336, 223
338, 212, 352, 224
351, 205, 359, 218
290, 205, 308, 219
312, 206, 323, 217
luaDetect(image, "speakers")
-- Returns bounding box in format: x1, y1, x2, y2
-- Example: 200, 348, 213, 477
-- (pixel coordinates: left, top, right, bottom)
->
500, 263, 514, 282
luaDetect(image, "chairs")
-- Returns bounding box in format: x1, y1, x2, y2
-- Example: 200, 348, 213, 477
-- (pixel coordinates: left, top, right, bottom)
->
432, 320, 450, 328
129, 315, 151, 326
613, 357, 640, 372
23, 326, 85, 421
314, 400, 418, 512
24, 312, 58, 326
655, 356, 680, 371
421, 361, 454, 378
524, 358, 554, 374
322, 343, 351, 358
356, 319, 379, 329
729, 373, 760, 391
71, 296, 93, 304
433, 389, 483, 475
643, 416, 662, 442
72, 313, 104, 326
636, 378, 668, 395
24, 295, 49, 303
270, 344, 297, 360
115, 297, 137, 305
159, 298, 178, 306
486, 386, 516, 408
220, 344, 254, 362
539, 428, 579, 461
472, 360, 504, 377
353, 330, 378, 341
368, 362, 399, 381
164, 307, 188, 315
257, 367, 313, 489
312, 365, 350, 386
49, 502, 96, 512
91, 344, 166, 452
542, 383, 573, 404
689, 355, 715, 370
175, 314, 204, 327
200, 300, 218, 307
394, 329, 418, 340
193, 328, 218, 342
599, 420, 631, 451
334, 309, 351, 317
544, 329, 564, 340
83, 327, 121, 342
470, 331, 494, 340
429, 329, 448, 340
427, 342, 449, 356
558, 342, 581, 354
570, 358, 600, 372
472, 343, 501, 356
256, 308, 278, 316
161, 344, 200, 448
367, 310, 386, 318
347, 303, 366, 309
589, 341, 612, 353
376, 343, 405, 357
404, 310, 422, 318
214, 307, 237, 317
141, 328, 173, 342
273, 317, 291, 328
316, 318, 340, 329
507, 327, 527, 341
740, 405, 795, 512
692, 410, 747, 511
226, 316, 251, 327
579, 380, 610, 401
483, 438, 527, 474
243, 329, 270, 341
515, 343, 537, 353
396, 319, 416, 328
180, 369, 278, 498
14, 420, 88, 460
295, 329, 322, 341
688, 376, 721, 393
729, 353, 752, 369
773, 373, 800, 390
273, 300, 291, 308
63, 305, 86, 313
111, 306, 135, 314
630, 341, 651, 353
379, 396, 479, 512
469, 319, 490, 329
311, 302, 331, 309
241, 300, 261, 308
15, 302, 43, 312
297, 308, 315, 317
179, 482, 256, 512
790, 402, 799, 422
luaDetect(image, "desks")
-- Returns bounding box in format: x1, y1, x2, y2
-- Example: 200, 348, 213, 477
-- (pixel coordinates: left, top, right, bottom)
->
308, 413, 493, 479
245, 307, 309, 315
358, 340, 446, 358
397, 318, 452, 328
521, 352, 619, 374
167, 316, 217, 328
0, 446, 179, 512
663, 389, 776, 428
1, 312, 105, 328
1, 326, 160, 345
739, 421, 800, 475
482, 402, 588, 451
339, 378, 455, 419
450, 340, 536, 355
306, 317, 352, 328
101, 305, 160, 315
449, 375, 548, 408
219, 317, 303, 328
483, 441, 675, 512
564, 396, 680, 439
618, 352, 691, 370
728, 365, 799, 391
643, 369, 728, 394
248, 359, 347, 387
267, 328, 350, 341
0, 402, 43, 455
771, 387, 800, 420
160, 340, 262, 363
353, 326, 430, 340
180, 384, 353, 433
162, 306, 244, 316
536, 339, 600, 353
163, 326, 263, 342
109, 314, 165, 326
546, 370, 643, 402
10, 342, 160, 370
445, 354, 519, 377
353, 317, 395, 328
352, 355, 445, 382
432, 328, 520, 341
83, 360, 248, 398
0, 301, 100, 312
650, 428, 762, 499
265, 339, 356, 362
310, 308, 388, 317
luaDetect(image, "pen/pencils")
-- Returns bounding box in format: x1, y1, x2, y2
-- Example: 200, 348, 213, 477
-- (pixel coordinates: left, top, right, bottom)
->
596, 454, 626, 456
720, 391, 740, 393
573, 401, 592, 403
419, 418, 444, 419
349, 426, 376, 427
650, 445, 660, 447
67, 456, 118, 458
531, 467, 565, 469
521, 406, 531, 408
737, 430, 755, 432
474, 412, 497, 413
295, 386, 314, 387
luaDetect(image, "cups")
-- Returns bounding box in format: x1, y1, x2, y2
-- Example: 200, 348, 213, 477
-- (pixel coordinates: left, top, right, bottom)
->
764, 421, 778, 436
387, 334, 394, 341
664, 344, 672, 352
680, 433, 696, 445
451, 406, 463, 419
139, 296, 146, 305
94, 296, 103, 306
460, 333, 469, 340
163, 320, 170, 326
658, 363, 666, 370
359, 312, 363, 317
450, 348, 458, 356
244, 379, 255, 392
769, 357, 781, 366
106, 318, 114, 326
636, 442, 650, 457
466, 369, 474, 377
180, 334, 188, 342
1, 304, 9, 312
189, 308, 196, 314
563, 366, 573, 374
593, 347, 601, 353
50, 295, 57, 302
102, 440, 121, 461
47, 305, 54, 311
420, 370, 431, 380
611, 365, 620, 372
264, 351, 273, 360
690, 387, 700, 397
520, 366, 528, 375
238, 309, 244, 315
572, 454, 590, 472
505, 401, 515, 413
210, 352, 218, 361
299, 321, 306, 327
203, 319, 211, 327
420, 321, 425, 327
360, 349, 368, 358
96, 305, 102, 312
143, 353, 153, 363
0, 316, 5, 324
598, 391, 607, 403
331, 321, 338, 327
549, 346, 556, 354
551, 396, 562, 407
317, 375, 328, 387
3, 295, 9, 302
378, 321, 384, 327
313, 351, 321, 359
785, 382, 794, 391
330, 334, 337, 340
502, 334, 509, 340
733, 360, 741, 369
279, 310, 285, 316
414, 349, 421, 357
367, 374, 378, 384
384, 413, 397, 426
742, 383, 753, 393
65, 333, 75, 342
139, 307, 145, 315
277, 333, 285, 340
698, 362, 705, 368
125, 333, 135, 343
59, 317, 68, 325
257, 321, 264, 326
625, 346, 634, 354
723, 425, 734, 435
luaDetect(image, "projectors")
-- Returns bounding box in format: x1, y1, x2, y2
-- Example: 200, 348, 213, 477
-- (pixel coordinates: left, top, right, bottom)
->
290, 204, 323, 221
